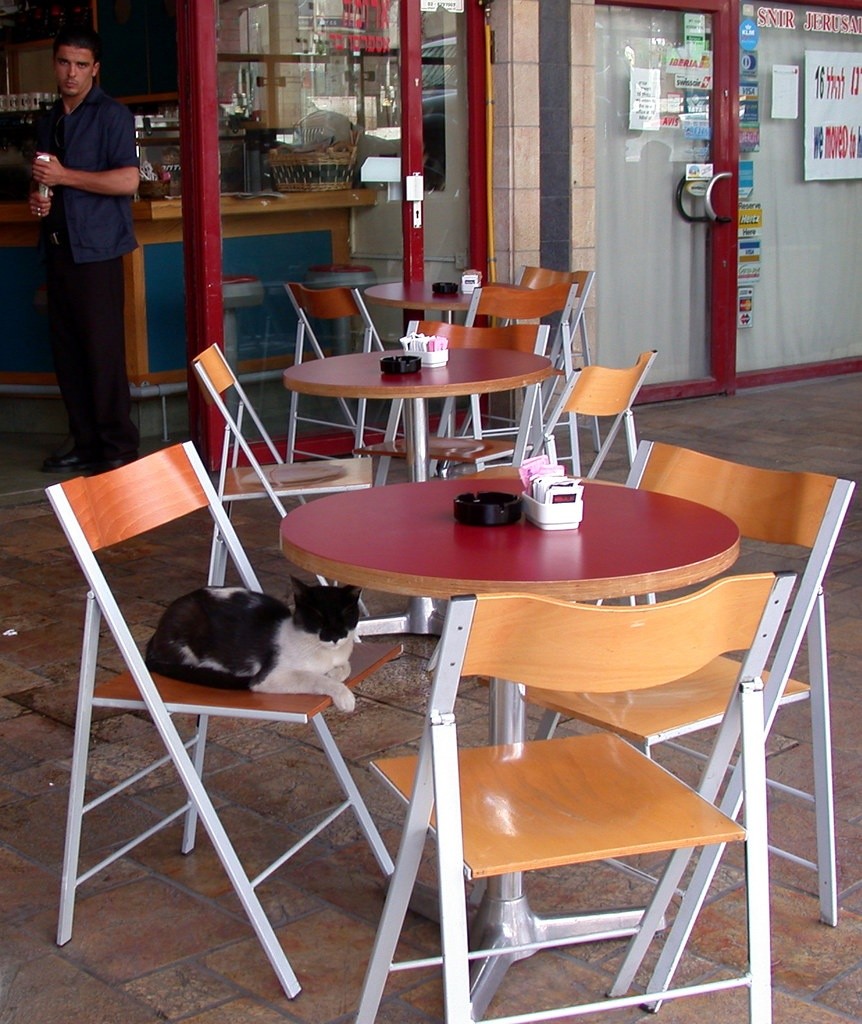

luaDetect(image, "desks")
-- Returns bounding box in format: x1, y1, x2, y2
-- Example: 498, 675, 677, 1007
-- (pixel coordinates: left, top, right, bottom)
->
279, 478, 740, 1024
283, 348, 554, 636
364, 282, 532, 479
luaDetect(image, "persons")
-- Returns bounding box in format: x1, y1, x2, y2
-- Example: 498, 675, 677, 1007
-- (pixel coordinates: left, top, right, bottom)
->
29, 20, 139, 473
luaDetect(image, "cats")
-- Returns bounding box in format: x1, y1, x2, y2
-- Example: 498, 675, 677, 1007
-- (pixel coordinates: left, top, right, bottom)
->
143, 573, 363, 714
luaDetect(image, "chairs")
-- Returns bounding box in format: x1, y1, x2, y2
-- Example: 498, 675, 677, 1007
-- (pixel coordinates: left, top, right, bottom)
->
46, 265, 856, 1024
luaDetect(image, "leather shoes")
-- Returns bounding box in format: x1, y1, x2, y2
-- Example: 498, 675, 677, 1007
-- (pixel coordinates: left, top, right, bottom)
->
93, 460, 124, 474
43, 449, 89, 471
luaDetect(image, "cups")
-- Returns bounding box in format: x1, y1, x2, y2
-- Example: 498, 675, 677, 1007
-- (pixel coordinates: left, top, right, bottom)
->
0, 93, 60, 111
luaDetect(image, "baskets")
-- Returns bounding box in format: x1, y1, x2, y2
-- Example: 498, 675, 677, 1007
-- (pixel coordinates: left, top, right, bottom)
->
266, 146, 353, 191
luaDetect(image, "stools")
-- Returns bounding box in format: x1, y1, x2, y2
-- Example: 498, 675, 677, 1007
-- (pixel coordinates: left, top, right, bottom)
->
304, 264, 376, 356
222, 275, 264, 383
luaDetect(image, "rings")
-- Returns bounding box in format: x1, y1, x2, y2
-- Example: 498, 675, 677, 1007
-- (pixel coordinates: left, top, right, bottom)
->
37, 207, 41, 212
38, 213, 41, 217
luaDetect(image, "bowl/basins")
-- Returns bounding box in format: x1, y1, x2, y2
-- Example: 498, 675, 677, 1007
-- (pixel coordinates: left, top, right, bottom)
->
522, 491, 584, 530
404, 349, 448, 367
461, 282, 482, 295
